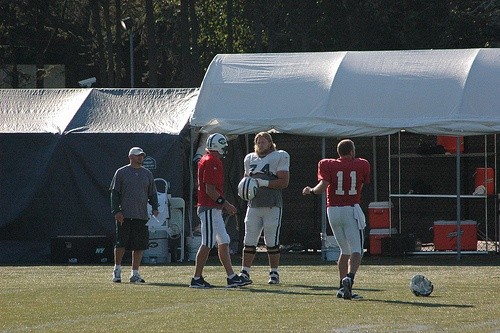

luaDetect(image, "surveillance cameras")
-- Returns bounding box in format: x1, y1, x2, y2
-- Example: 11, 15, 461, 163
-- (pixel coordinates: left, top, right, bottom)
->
77, 77, 97, 88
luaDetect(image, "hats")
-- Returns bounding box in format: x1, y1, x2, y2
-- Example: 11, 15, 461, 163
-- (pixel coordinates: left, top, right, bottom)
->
128, 147, 146, 158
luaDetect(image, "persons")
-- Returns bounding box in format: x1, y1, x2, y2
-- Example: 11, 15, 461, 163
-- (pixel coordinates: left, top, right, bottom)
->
189, 134, 252, 288
238, 131, 289, 284
303, 139, 371, 298
109, 147, 160, 282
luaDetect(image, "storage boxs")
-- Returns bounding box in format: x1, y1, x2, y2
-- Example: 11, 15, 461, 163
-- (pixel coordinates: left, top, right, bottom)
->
368, 201, 394, 229
370, 227, 399, 254
433, 220, 478, 249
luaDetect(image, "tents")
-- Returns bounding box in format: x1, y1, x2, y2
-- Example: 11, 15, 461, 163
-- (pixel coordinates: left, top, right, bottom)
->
0, 48, 500, 264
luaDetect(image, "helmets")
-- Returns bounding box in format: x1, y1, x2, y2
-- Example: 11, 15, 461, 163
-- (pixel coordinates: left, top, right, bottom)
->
409, 274, 433, 296
237, 177, 258, 201
206, 133, 228, 155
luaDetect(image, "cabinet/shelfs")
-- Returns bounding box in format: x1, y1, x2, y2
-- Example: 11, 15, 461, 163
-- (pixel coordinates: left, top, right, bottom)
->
388, 129, 500, 255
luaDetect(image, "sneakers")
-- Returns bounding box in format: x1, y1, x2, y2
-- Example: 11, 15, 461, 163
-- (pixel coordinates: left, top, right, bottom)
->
129, 273, 145, 283
238, 270, 250, 280
336, 287, 363, 299
227, 274, 253, 287
112, 267, 122, 282
267, 271, 279, 284
189, 275, 211, 288
342, 274, 353, 300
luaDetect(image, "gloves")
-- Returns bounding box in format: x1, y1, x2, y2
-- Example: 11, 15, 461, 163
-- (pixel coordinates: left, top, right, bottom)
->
255, 178, 269, 188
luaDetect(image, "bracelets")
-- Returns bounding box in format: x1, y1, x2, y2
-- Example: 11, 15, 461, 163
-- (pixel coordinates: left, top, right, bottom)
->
310, 188, 315, 195
217, 197, 225, 205
